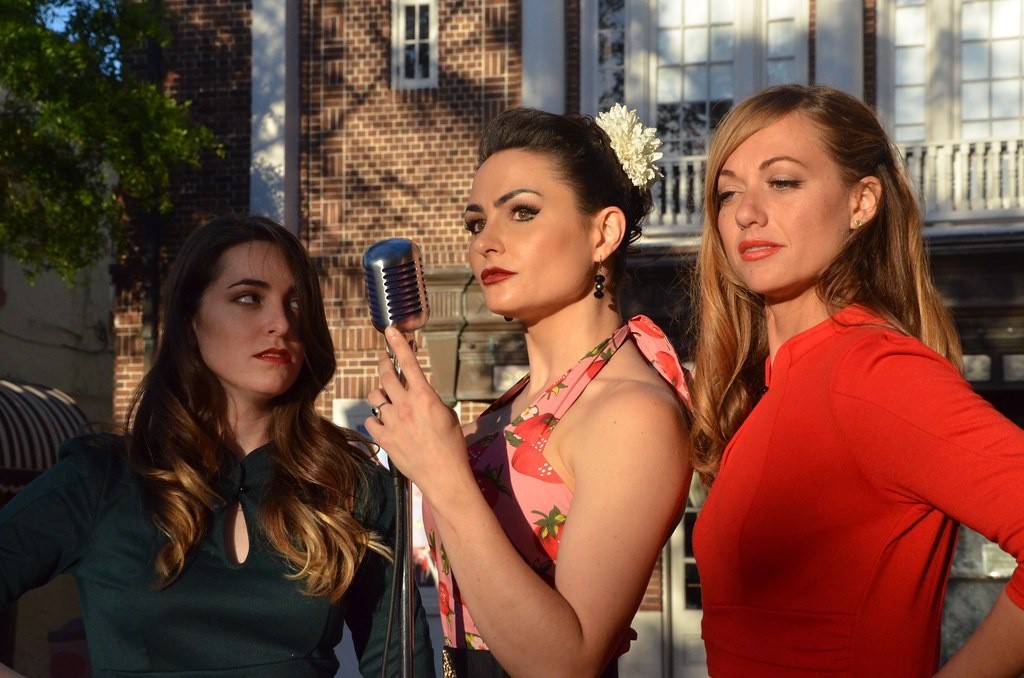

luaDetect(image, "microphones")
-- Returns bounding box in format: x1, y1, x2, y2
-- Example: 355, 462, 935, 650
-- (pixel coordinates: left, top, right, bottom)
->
362, 236, 431, 358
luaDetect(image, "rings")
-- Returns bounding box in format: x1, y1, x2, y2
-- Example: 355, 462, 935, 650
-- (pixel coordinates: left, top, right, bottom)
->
372, 402, 389, 417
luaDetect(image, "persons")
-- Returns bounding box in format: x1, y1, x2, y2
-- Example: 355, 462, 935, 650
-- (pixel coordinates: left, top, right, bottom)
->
684, 84, 1024, 678
366, 109, 697, 678
0, 216, 433, 677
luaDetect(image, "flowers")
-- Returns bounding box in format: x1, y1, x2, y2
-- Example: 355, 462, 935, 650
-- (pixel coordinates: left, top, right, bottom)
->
594, 102, 666, 193
